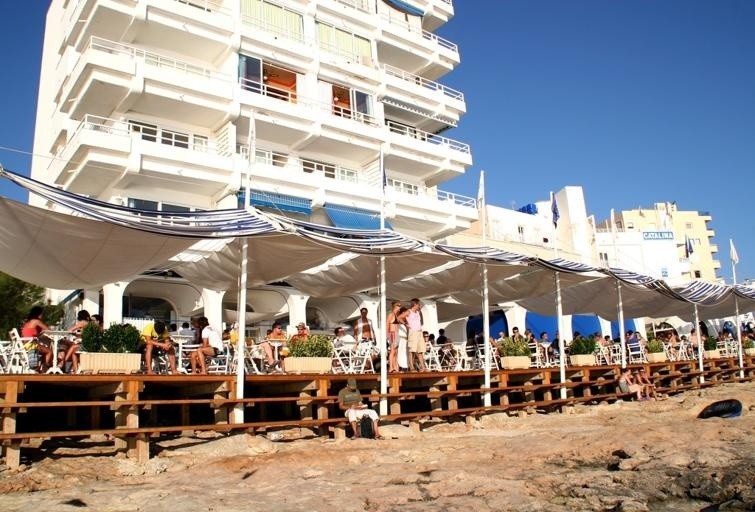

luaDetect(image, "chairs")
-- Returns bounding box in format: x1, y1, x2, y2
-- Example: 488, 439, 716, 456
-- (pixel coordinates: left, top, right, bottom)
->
332, 334, 499, 374
662, 340, 706, 362
140, 332, 286, 376
1, 321, 83, 374
716, 338, 744, 357
594, 339, 648, 365
528, 341, 570, 367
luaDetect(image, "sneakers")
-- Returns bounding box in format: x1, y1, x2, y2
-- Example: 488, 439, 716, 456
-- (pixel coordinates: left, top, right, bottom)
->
268, 360, 282, 375
409, 367, 429, 372
375, 435, 385, 440
636, 396, 651, 401
351, 436, 357, 440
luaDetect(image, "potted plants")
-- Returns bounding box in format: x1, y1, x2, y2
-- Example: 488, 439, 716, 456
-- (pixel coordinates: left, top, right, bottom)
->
285, 334, 334, 375
744, 339, 755, 356
568, 338, 599, 366
76, 319, 145, 374
646, 338, 665, 363
704, 337, 719, 359
497, 336, 530, 370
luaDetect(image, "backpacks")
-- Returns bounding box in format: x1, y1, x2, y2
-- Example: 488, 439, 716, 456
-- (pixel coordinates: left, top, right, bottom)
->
360, 414, 375, 439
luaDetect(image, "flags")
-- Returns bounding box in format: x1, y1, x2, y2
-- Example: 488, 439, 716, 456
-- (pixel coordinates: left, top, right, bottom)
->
550, 190, 560, 230
610, 207, 619, 244
380, 144, 388, 197
685, 230, 694, 260
246, 107, 257, 173
476, 169, 485, 210
729, 238, 739, 265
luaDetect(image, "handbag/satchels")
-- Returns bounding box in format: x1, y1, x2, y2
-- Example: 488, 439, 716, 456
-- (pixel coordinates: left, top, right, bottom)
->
28, 349, 39, 369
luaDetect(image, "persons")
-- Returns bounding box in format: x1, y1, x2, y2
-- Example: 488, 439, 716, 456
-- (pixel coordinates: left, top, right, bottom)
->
334, 307, 379, 371
387, 298, 458, 373
223, 320, 309, 372
656, 328, 706, 360
466, 326, 570, 371
56, 313, 104, 369
632, 367, 666, 402
58, 309, 93, 374
711, 323, 754, 352
21, 305, 53, 376
619, 367, 647, 402
338, 376, 386, 441
142, 317, 224, 374
573, 331, 647, 365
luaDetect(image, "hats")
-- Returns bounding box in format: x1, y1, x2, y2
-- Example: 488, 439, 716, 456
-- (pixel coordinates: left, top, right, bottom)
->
347, 378, 356, 390
296, 323, 306, 329
